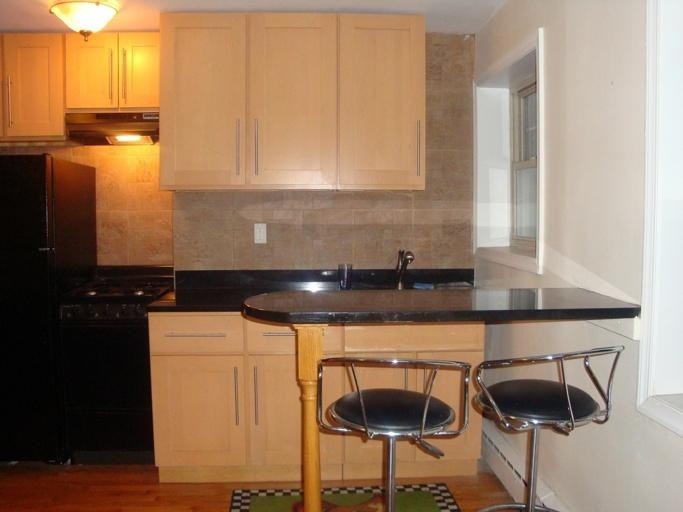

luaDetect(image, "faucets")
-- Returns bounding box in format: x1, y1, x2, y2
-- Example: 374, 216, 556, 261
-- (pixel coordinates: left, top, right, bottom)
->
398, 251, 414, 280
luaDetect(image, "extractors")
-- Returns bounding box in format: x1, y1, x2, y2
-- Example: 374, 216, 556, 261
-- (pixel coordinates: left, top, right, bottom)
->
65, 119, 160, 148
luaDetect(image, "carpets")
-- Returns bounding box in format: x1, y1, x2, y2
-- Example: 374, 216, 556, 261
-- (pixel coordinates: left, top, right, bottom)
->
227, 476, 459, 511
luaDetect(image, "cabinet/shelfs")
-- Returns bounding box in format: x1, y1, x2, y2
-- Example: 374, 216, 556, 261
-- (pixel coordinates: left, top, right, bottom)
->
338, 11, 426, 195
144, 311, 344, 485
158, 10, 338, 194
344, 322, 486, 481
64, 31, 159, 114
0, 29, 64, 144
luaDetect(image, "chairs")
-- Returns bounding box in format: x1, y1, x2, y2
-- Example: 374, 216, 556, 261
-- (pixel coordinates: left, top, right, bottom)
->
472, 343, 628, 510
313, 353, 470, 512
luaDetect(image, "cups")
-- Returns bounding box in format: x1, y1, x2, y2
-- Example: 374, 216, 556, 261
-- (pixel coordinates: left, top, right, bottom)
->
338, 262, 352, 289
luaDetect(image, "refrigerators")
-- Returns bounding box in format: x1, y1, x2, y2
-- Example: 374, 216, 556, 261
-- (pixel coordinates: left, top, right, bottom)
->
0, 150, 97, 466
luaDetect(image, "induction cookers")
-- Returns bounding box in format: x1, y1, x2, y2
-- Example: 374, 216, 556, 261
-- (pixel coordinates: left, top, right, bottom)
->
57, 282, 173, 325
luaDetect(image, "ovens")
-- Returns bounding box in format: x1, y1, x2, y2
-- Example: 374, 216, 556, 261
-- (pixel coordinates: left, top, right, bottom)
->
57, 321, 155, 465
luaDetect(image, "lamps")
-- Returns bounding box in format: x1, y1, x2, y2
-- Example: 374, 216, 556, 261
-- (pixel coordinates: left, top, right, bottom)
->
47, 2, 123, 43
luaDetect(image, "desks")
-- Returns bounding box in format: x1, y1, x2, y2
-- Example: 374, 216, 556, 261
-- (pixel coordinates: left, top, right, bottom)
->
238, 285, 642, 510
